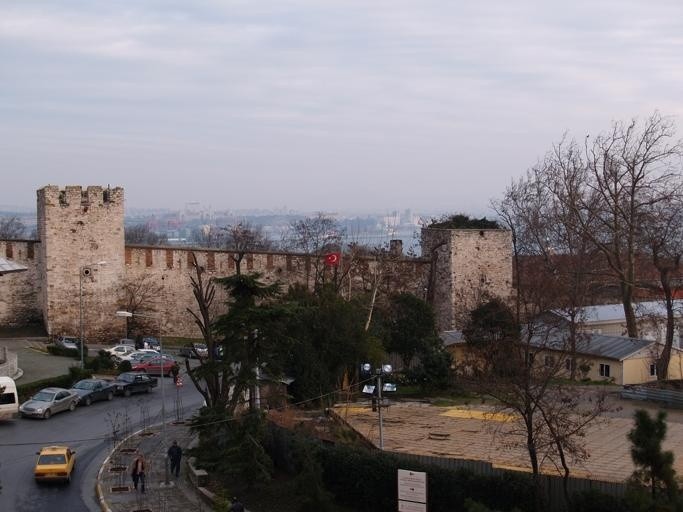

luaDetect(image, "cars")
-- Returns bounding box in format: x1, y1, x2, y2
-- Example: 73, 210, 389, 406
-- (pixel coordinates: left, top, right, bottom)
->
131, 357, 179, 377
54, 335, 78, 351
214, 343, 223, 359
67, 374, 115, 406
33, 446, 76, 486
178, 342, 208, 359
102, 337, 173, 368
107, 371, 157, 397
18, 387, 80, 420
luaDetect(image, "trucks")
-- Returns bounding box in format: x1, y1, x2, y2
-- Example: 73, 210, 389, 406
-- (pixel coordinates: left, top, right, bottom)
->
0, 376, 18, 421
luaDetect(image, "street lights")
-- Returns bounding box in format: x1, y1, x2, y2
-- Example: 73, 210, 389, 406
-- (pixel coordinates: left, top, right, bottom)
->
539, 211, 555, 310
360, 363, 397, 451
116, 310, 166, 444
80, 260, 106, 370
243, 328, 267, 420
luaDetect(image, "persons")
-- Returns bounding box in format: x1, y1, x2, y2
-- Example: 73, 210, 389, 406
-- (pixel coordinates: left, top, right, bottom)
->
169, 359, 180, 384
147, 341, 153, 350
131, 455, 148, 495
141, 339, 147, 350
167, 439, 183, 478
226, 496, 244, 511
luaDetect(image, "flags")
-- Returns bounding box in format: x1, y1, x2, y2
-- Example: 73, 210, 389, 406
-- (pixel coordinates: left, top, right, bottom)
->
323, 250, 342, 267
